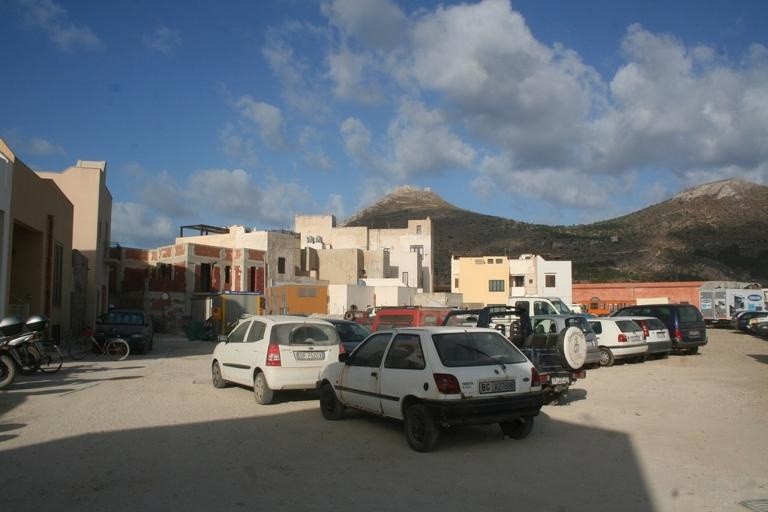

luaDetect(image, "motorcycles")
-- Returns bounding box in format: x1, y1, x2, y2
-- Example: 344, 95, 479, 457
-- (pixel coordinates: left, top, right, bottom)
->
0, 313, 49, 389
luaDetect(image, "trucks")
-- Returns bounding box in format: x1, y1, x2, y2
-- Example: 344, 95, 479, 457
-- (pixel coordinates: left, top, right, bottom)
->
699, 280, 768, 329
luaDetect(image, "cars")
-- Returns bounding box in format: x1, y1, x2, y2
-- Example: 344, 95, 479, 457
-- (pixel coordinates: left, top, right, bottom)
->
93, 309, 153, 354
731, 309, 768, 337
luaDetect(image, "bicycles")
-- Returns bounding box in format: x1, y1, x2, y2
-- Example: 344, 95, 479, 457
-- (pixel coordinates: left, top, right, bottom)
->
39, 320, 130, 374
